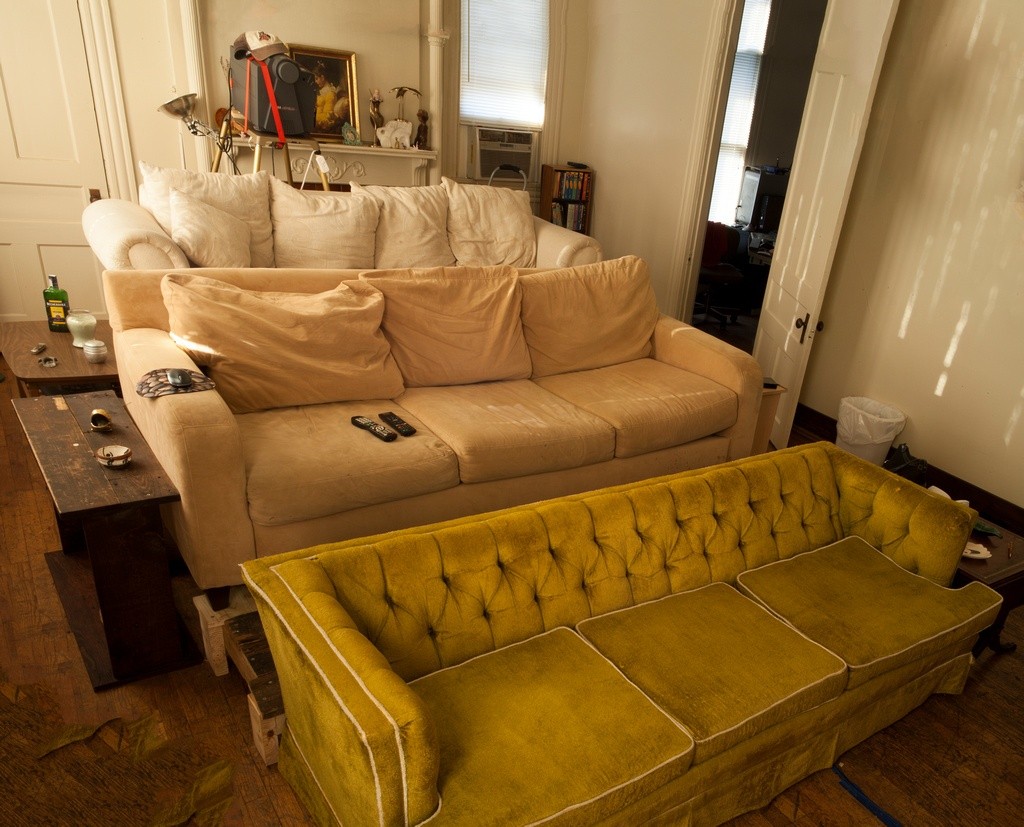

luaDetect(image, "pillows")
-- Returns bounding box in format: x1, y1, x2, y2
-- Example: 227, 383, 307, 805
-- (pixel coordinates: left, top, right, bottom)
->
169, 189, 253, 269
161, 274, 405, 414
138, 161, 274, 269
350, 180, 459, 270
359, 266, 532, 388
440, 175, 536, 268
519, 255, 658, 380
269, 173, 383, 270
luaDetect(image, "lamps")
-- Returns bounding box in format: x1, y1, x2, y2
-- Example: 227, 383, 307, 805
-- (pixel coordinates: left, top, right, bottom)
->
158, 93, 241, 175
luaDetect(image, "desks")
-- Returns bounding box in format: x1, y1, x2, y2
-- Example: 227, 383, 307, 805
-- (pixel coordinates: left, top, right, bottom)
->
954, 513, 1024, 661
0, 317, 123, 399
12, 391, 205, 691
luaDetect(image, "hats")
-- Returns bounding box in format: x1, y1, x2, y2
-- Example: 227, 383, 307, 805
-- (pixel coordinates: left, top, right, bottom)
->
234, 32, 289, 59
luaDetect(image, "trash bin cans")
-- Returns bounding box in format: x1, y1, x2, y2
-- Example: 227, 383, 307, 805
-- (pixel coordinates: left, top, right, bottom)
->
835, 397, 906, 466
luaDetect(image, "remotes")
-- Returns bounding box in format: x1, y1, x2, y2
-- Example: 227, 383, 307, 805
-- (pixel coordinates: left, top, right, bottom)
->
378, 412, 417, 436
350, 416, 398, 443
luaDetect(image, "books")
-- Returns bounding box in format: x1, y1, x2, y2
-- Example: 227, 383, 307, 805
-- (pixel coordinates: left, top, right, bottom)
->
553, 171, 590, 198
551, 202, 586, 232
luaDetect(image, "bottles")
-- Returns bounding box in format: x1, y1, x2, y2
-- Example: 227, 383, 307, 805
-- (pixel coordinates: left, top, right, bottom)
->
42, 275, 72, 332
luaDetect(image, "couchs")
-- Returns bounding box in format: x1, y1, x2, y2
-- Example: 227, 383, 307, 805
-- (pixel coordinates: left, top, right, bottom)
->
106, 261, 762, 591
81, 180, 600, 270
236, 439, 1003, 826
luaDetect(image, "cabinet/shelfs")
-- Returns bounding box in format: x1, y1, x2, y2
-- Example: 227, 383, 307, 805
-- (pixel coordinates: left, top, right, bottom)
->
540, 163, 592, 236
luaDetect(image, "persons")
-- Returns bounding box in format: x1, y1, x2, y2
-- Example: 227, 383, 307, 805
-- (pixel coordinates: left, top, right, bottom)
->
313, 64, 338, 130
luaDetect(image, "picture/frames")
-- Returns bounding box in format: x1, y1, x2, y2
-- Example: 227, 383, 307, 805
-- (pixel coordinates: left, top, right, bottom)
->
286, 43, 360, 143
